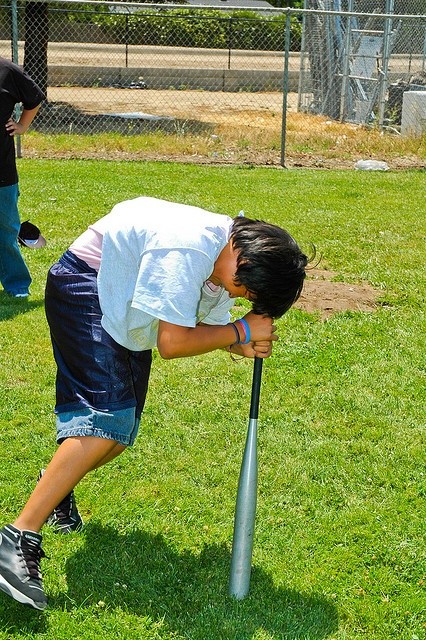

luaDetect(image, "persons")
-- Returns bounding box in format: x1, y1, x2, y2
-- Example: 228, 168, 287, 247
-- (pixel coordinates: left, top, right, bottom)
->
0, 196, 323, 610
0, 55, 46, 298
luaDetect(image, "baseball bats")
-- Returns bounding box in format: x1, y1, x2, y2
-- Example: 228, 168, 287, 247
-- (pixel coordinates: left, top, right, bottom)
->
226, 358, 264, 601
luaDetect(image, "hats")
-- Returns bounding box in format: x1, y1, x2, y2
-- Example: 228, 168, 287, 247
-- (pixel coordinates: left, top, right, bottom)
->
19, 220, 49, 252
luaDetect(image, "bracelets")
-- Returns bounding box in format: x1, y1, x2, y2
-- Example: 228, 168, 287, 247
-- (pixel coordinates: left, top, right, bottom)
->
227, 323, 245, 362
235, 318, 250, 344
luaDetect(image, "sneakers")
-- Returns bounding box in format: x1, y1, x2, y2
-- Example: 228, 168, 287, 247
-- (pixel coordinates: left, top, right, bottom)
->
37, 470, 85, 537
0, 521, 49, 612
